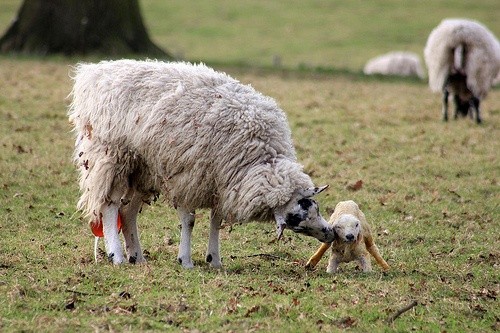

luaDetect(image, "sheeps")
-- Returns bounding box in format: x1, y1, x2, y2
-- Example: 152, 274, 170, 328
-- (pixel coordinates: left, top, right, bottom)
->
67, 57, 336, 271
305, 200, 391, 276
424, 18, 500, 124
364, 51, 425, 80
